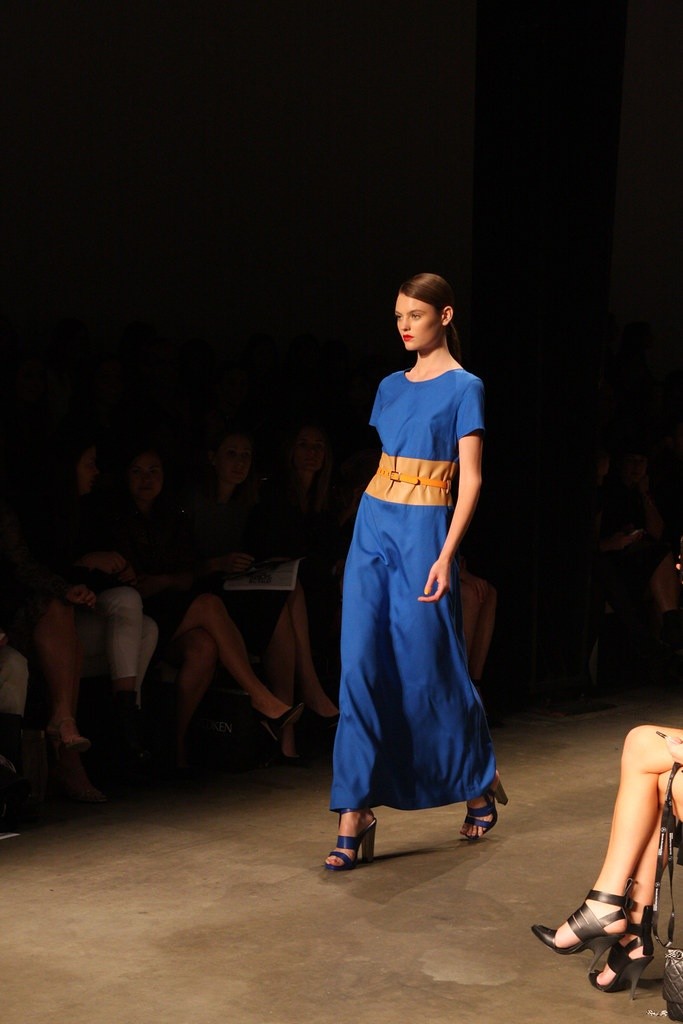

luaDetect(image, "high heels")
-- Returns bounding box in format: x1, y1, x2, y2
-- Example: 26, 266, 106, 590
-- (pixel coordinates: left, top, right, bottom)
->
588, 906, 654, 999
55, 718, 91, 760
249, 704, 305, 742
532, 890, 630, 969
68, 786, 106, 802
464, 780, 508, 839
326, 808, 376, 869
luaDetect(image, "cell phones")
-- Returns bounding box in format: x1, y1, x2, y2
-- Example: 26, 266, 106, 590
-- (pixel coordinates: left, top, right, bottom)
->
629, 529, 644, 536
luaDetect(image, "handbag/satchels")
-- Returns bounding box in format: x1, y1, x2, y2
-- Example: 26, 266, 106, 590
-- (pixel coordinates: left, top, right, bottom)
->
663, 948, 683, 1022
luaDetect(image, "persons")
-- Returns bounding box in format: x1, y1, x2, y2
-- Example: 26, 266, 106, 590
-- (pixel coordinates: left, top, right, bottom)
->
1, 411, 683, 800
530, 725, 683, 1001
323, 274, 509, 872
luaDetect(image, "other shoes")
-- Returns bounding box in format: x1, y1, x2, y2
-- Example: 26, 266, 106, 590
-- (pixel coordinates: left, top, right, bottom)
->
105, 759, 150, 785
278, 750, 306, 766
0, 802, 15, 833
307, 707, 342, 727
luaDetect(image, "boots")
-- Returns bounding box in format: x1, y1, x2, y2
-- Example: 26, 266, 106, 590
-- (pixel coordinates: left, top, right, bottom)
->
0, 713, 31, 803
113, 690, 146, 760
659, 610, 683, 656
